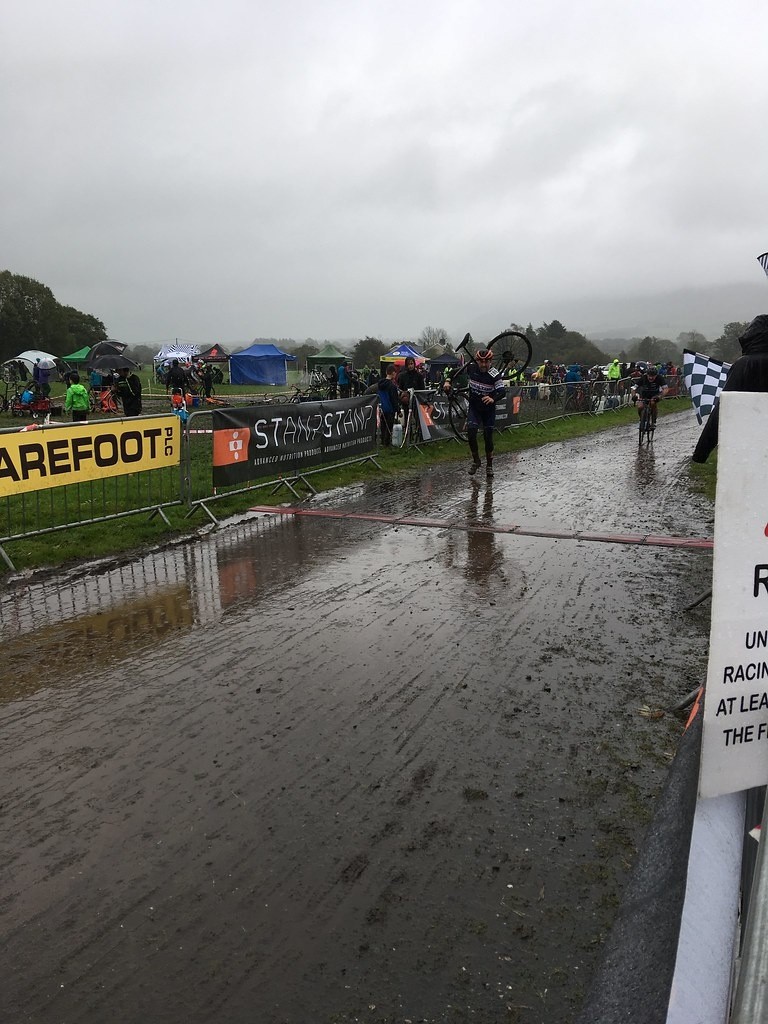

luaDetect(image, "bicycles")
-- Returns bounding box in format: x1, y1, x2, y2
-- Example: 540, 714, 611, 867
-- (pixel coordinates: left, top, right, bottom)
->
591, 382, 619, 412
81, 384, 132, 417
166, 380, 218, 403
634, 396, 660, 446
424, 330, 534, 441
245, 392, 288, 408
289, 380, 342, 405
559, 386, 592, 414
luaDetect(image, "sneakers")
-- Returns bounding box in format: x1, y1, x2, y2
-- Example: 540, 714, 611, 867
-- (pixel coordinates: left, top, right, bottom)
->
486, 465, 494, 479
651, 422, 658, 431
468, 460, 483, 477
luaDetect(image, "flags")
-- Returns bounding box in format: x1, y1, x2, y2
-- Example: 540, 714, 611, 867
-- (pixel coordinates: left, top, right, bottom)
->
682, 348, 734, 426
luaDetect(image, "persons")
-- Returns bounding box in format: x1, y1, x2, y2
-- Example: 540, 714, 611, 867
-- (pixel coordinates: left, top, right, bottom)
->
32, 355, 685, 476
692, 314, 768, 463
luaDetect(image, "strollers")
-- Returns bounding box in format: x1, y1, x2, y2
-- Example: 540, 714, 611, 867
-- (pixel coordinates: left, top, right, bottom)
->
12, 381, 53, 418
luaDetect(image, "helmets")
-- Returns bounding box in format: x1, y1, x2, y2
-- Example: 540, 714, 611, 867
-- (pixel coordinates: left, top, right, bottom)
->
198, 358, 205, 365
646, 368, 659, 375
476, 349, 493, 360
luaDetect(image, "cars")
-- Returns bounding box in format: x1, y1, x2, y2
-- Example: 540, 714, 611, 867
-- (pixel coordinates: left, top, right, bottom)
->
505, 359, 642, 382
